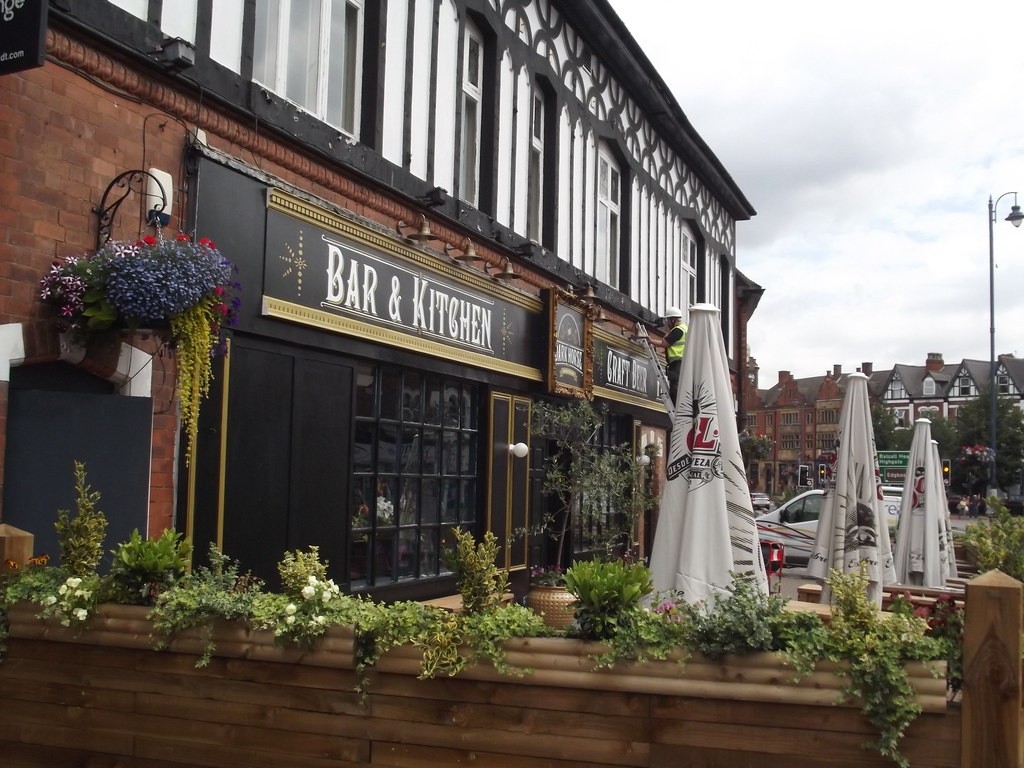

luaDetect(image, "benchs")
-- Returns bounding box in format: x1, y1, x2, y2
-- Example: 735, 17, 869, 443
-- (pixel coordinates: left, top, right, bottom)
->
782, 570, 978, 640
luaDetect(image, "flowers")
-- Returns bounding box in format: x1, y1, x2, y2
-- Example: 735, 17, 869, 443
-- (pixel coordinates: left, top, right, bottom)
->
278, 571, 344, 639
650, 600, 686, 629
39, 231, 245, 474
39, 575, 93, 629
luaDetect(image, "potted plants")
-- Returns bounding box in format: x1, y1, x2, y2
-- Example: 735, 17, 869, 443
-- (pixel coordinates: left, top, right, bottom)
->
503, 393, 662, 634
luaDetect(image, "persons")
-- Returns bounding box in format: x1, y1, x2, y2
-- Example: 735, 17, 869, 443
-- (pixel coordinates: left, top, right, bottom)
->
645, 307, 690, 409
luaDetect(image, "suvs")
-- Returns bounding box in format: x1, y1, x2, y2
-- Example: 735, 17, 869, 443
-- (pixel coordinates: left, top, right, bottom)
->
749, 492, 771, 510
748, 486, 905, 571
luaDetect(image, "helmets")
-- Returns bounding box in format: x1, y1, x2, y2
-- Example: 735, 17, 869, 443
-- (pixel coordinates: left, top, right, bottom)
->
664, 307, 682, 318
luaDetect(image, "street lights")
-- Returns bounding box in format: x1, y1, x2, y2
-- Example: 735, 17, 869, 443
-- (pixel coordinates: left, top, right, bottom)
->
987, 191, 1024, 524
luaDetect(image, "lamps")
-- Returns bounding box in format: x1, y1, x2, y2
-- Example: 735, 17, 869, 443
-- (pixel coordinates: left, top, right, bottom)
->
565, 280, 602, 307
621, 323, 649, 344
586, 304, 613, 325
484, 255, 523, 284
396, 213, 441, 247
446, 235, 484, 267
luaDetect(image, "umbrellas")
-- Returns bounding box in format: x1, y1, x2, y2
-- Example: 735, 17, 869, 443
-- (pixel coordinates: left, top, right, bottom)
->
894, 416, 950, 589
805, 372, 897, 612
646, 304, 774, 616
929, 439, 959, 581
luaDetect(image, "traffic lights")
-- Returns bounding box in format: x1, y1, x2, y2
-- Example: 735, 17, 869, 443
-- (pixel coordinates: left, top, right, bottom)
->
819, 464, 826, 483
942, 458, 951, 486
798, 465, 808, 487
